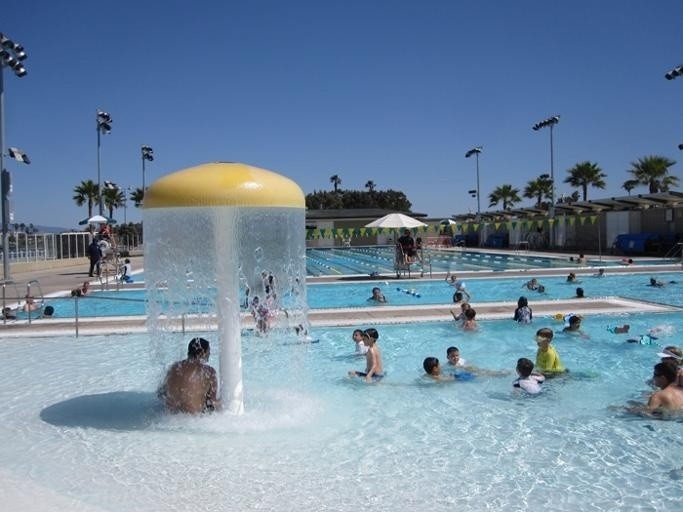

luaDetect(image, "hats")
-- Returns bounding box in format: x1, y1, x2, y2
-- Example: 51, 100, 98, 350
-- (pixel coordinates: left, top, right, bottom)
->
656, 350, 683, 360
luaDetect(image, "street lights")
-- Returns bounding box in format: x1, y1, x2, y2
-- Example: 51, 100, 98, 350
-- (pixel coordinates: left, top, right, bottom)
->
122, 186, 134, 225
94, 107, 112, 215
665, 63, 682, 82
465, 144, 482, 215
531, 116, 560, 206
0, 32, 27, 284
139, 144, 155, 195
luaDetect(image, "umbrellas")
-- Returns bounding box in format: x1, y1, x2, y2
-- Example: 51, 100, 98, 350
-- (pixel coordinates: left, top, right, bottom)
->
79, 215, 117, 225
364, 213, 429, 244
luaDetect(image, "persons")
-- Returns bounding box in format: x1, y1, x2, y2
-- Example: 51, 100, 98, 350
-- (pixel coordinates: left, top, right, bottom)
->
0, 294, 54, 319
648, 346, 683, 411
245, 274, 276, 331
513, 327, 560, 392
295, 325, 314, 344
371, 287, 385, 302
525, 254, 676, 346
399, 230, 422, 263
513, 295, 533, 325
86, 230, 131, 281
445, 272, 476, 328
71, 281, 89, 299
423, 347, 460, 373
157, 338, 218, 417
353, 328, 383, 377
